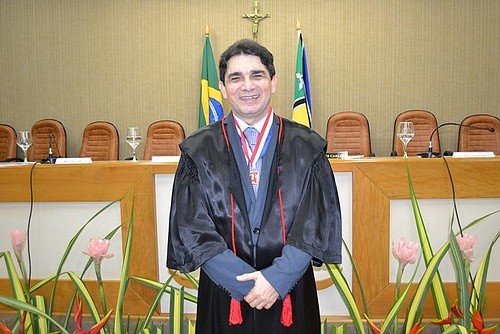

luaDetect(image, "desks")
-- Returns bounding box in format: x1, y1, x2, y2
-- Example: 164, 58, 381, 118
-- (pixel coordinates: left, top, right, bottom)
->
0, 162, 500, 319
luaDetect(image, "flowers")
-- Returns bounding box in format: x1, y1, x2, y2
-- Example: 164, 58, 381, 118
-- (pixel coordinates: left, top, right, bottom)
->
0, 166, 500, 334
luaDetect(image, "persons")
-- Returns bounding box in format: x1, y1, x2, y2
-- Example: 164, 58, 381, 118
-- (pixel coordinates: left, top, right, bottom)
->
245, 13, 269, 40
165, 38, 342, 334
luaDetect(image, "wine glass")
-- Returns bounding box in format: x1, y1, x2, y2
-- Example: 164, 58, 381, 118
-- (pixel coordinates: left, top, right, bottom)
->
397, 121, 415, 158
125, 127, 142, 162
16, 131, 33, 164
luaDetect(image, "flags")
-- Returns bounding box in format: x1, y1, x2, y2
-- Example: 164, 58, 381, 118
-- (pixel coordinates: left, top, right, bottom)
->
291, 33, 312, 127
198, 38, 224, 128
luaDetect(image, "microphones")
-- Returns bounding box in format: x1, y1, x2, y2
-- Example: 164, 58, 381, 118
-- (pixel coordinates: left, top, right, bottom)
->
41, 133, 63, 164
416, 123, 494, 158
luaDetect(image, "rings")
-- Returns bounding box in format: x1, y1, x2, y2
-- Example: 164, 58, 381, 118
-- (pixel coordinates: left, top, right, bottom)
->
259, 303, 263, 308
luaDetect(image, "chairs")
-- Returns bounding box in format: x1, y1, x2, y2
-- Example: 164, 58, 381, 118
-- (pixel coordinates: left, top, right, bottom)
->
0, 114, 19, 163
77, 118, 123, 160
391, 108, 443, 158
455, 112, 500, 154
325, 110, 372, 158
22, 116, 70, 163
143, 119, 190, 162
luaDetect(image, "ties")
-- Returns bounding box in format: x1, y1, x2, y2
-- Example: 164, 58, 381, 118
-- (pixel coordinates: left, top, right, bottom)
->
244, 128, 260, 150
